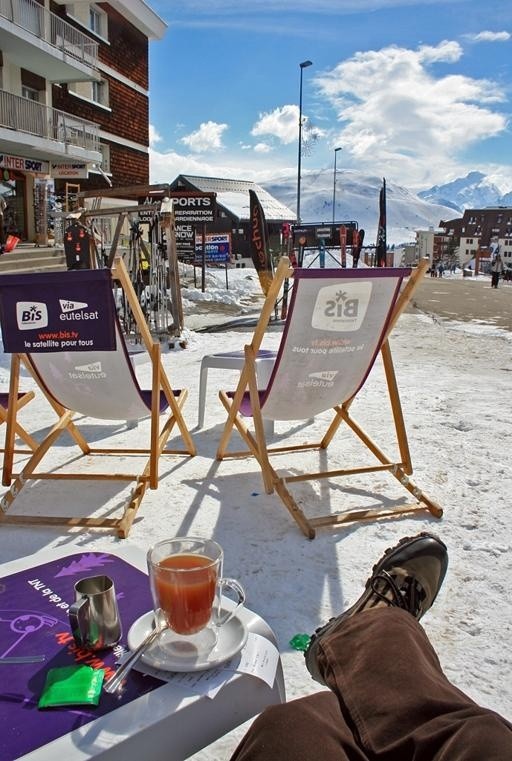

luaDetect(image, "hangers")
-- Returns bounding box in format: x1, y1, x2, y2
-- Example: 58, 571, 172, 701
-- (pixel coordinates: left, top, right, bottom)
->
103, 607, 170, 695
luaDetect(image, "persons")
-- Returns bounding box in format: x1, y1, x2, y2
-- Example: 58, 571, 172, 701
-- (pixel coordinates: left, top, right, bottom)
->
430, 264, 436, 277
438, 265, 444, 278
489, 253, 506, 288
229, 536, 512, 761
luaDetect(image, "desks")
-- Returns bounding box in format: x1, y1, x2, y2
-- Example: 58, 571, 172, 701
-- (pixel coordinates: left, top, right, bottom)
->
297, 60, 312, 226
332, 147, 342, 224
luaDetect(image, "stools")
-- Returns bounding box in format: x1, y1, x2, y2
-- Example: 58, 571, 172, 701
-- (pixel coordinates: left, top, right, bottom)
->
198, 349, 315, 437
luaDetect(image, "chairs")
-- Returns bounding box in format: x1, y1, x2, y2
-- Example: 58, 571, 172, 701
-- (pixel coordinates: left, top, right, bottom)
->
0, 256, 198, 538
215, 254, 443, 540
306, 532, 448, 686
0, 389, 39, 486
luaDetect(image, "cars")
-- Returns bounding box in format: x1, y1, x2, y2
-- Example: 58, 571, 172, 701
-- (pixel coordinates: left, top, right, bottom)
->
68, 574, 123, 651
145, 534, 247, 659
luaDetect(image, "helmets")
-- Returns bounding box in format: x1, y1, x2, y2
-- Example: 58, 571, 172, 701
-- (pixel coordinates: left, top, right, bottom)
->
126, 606, 250, 674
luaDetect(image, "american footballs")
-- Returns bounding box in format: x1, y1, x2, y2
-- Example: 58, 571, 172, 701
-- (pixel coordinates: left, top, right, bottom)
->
62, 223, 91, 269
